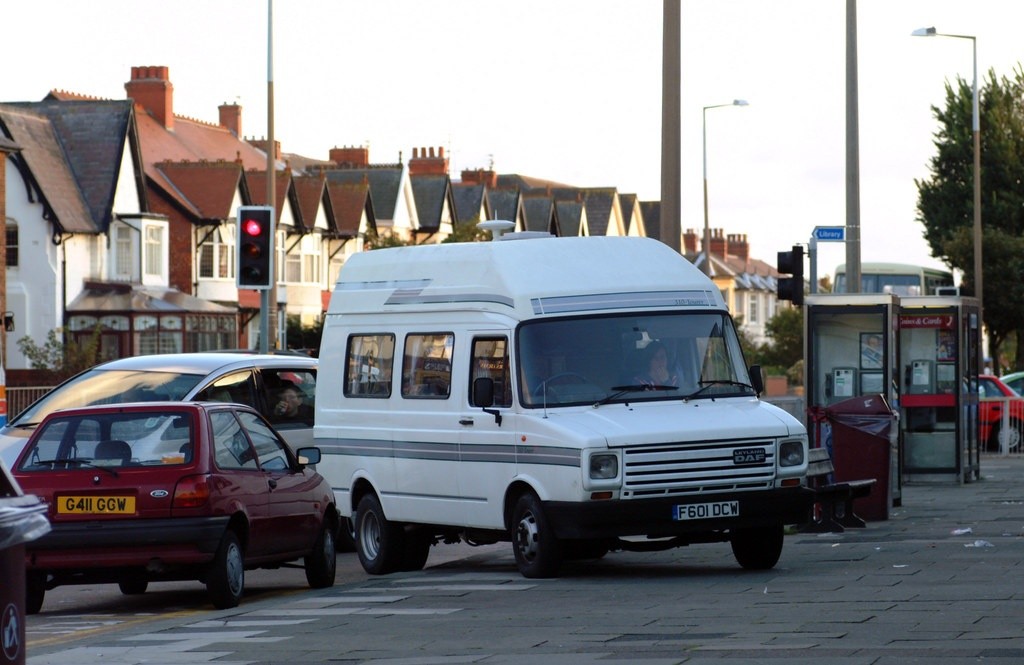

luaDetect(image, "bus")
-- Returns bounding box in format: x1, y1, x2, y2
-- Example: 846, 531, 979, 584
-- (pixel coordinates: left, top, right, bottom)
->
832, 263, 955, 297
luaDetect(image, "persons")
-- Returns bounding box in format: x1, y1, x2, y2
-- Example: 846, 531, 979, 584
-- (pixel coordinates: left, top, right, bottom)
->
939, 342, 947, 353
634, 344, 680, 390
984, 358, 994, 376
862, 335, 881, 365
267, 385, 314, 425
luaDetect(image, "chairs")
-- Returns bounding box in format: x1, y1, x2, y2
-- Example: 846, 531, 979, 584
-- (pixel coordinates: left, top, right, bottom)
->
423, 375, 446, 397
617, 346, 687, 388
180, 444, 220, 466
494, 382, 506, 406
534, 348, 596, 396
93, 440, 143, 466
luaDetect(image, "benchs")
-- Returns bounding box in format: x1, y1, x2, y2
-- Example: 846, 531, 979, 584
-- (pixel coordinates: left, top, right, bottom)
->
784, 446, 879, 531
346, 379, 392, 397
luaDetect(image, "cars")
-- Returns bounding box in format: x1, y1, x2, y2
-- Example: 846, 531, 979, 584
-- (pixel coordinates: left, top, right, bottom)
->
0, 353, 381, 554
10, 401, 338, 615
978, 374, 1024, 453
978, 372, 1024, 399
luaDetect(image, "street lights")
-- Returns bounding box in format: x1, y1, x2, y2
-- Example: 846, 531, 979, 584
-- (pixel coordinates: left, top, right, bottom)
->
703, 99, 749, 279
911, 26, 983, 327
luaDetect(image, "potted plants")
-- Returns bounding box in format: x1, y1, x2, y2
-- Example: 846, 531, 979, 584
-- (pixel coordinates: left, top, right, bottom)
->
766, 365, 791, 398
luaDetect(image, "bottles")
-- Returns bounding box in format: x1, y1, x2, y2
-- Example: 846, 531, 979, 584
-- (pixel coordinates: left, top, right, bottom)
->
277, 401, 289, 416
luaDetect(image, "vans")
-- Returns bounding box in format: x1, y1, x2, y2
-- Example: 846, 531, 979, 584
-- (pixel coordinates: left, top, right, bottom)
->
311, 236, 811, 578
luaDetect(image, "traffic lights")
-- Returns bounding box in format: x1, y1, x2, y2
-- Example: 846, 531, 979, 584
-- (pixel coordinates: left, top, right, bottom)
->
237, 205, 275, 289
777, 246, 804, 305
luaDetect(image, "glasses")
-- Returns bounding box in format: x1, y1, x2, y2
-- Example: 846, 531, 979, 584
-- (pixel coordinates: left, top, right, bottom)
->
278, 394, 299, 402
